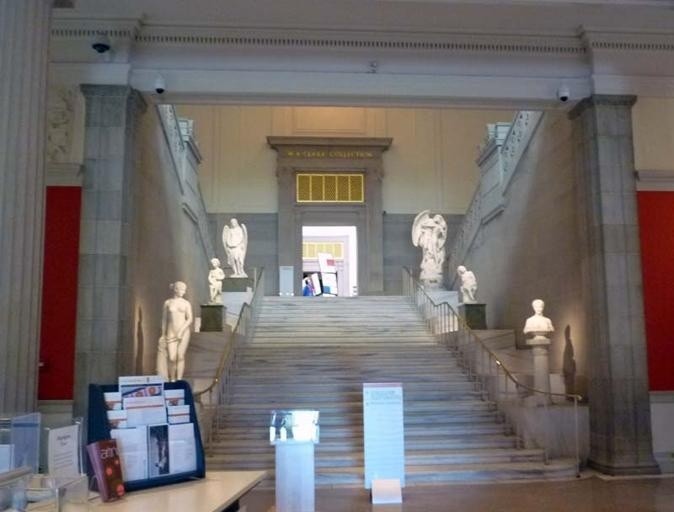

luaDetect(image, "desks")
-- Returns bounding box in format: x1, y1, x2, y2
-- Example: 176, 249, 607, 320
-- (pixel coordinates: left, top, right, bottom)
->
4, 470, 268, 512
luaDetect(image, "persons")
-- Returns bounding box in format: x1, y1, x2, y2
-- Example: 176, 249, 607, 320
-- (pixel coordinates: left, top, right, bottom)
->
221, 218, 249, 277
454, 264, 479, 302
207, 257, 223, 301
156, 282, 194, 379
411, 210, 447, 270
522, 297, 555, 336
